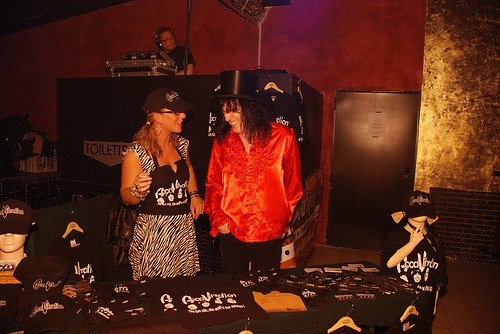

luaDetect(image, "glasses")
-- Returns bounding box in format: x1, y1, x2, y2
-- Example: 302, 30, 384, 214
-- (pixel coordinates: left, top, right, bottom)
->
157, 109, 181, 115
160, 37, 174, 42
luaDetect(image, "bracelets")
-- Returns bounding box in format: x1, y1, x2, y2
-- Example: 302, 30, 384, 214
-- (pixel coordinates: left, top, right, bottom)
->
189, 190, 201, 200
130, 183, 150, 201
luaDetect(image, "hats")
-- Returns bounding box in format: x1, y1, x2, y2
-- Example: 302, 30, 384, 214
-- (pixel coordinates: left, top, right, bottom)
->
13, 255, 69, 291
406, 190, 436, 218
24, 292, 71, 334
0, 199, 39, 234
207, 69, 268, 104
144, 88, 195, 112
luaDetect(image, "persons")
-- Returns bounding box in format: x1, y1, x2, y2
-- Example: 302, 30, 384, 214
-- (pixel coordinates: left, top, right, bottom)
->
120, 88, 205, 280
203, 70, 303, 272
0, 200, 39, 272
154, 28, 195, 75
383, 191, 448, 298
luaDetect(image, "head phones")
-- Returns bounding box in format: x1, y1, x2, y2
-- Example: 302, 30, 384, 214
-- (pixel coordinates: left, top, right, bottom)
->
155, 28, 176, 49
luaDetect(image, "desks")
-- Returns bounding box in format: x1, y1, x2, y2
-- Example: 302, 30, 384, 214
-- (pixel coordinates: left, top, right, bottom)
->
0, 261, 426, 334
